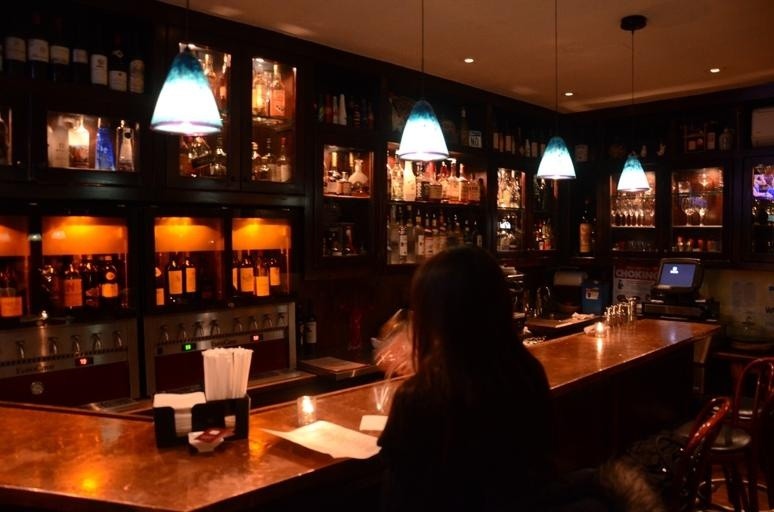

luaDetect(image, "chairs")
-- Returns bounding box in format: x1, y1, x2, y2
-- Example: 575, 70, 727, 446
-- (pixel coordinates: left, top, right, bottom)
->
538, 354, 774, 512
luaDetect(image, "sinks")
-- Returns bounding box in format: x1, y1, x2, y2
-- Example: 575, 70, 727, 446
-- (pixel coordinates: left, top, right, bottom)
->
541, 312, 573, 320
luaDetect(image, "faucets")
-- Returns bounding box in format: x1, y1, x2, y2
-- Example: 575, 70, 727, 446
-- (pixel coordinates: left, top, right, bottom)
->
536, 283, 551, 315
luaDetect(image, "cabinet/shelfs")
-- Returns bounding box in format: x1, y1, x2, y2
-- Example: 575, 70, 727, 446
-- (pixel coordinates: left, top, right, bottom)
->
313, 40, 568, 358
0, 0, 312, 393
567, 76, 773, 275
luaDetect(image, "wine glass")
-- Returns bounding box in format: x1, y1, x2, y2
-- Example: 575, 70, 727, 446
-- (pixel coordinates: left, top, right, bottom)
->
610, 168, 713, 227
605, 297, 636, 329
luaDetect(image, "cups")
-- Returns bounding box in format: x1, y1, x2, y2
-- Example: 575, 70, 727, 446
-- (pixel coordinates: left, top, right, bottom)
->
371, 308, 417, 372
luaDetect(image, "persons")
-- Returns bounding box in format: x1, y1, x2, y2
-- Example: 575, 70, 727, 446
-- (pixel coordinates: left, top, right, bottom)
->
376, 245, 553, 512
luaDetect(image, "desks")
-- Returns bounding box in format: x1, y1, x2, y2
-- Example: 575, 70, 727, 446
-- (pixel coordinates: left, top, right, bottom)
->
633, 300, 720, 397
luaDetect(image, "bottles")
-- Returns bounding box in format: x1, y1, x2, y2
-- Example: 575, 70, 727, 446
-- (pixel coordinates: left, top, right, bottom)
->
296, 303, 305, 346
579, 201, 593, 255
180, 53, 290, 183
2, 26, 149, 170
318, 94, 555, 263
0, 248, 295, 323
683, 114, 734, 156
305, 303, 317, 347
573, 143, 588, 163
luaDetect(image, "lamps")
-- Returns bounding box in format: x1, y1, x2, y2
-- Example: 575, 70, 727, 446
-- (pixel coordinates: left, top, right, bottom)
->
535, 0, 577, 182
151, 0, 223, 136
400, 0, 448, 165
617, 13, 650, 192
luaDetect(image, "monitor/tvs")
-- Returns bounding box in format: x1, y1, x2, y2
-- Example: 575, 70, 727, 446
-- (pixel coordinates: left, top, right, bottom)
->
651, 257, 705, 304
